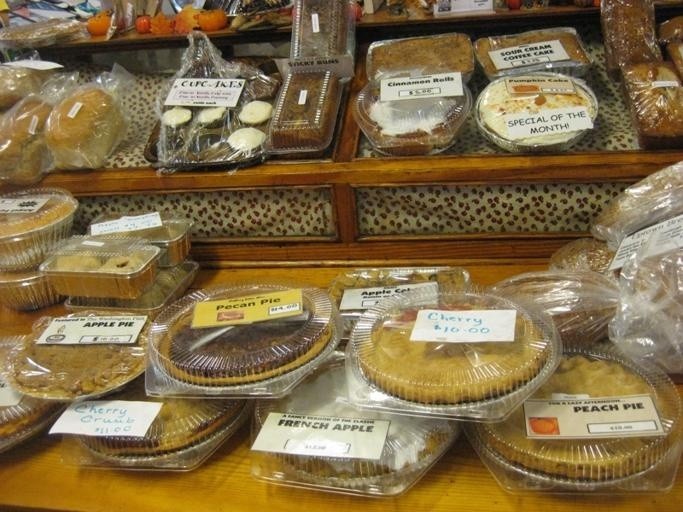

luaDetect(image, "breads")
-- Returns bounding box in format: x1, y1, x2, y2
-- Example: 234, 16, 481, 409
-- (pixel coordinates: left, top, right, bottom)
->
70, 389, 232, 465
0, 332, 65, 451
155, 282, 325, 392
476, 332, 677, 492
0, 186, 199, 314
251, 345, 454, 492
333, 259, 473, 327
1, 0, 683, 184
13, 310, 152, 396
494, 163, 682, 369
347, 281, 557, 423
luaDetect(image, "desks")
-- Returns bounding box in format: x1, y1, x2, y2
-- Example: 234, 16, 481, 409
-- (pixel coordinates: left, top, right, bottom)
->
1, 265, 682, 511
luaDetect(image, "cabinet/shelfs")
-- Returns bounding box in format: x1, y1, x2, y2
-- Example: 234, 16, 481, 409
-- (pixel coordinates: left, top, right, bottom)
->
0, 0, 681, 260
